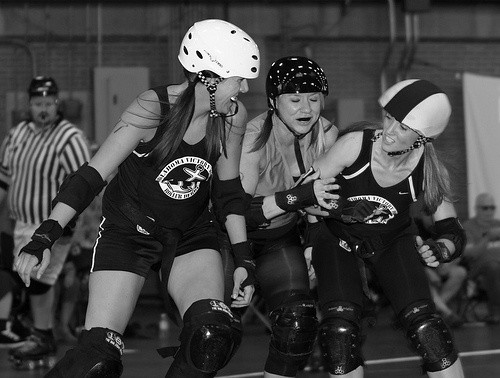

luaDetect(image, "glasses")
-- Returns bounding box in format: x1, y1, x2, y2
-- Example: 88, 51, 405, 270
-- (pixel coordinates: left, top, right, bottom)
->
476, 205, 496, 211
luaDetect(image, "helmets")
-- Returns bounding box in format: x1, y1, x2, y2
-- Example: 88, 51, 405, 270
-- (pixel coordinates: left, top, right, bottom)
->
378, 78, 452, 140
27, 75, 59, 99
265, 55, 329, 98
177, 18, 260, 80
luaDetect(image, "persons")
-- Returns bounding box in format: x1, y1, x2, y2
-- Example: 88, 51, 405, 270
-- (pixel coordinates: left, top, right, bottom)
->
426, 194, 500, 330
210, 56, 340, 378
289, 79, 467, 378
0, 75, 102, 370
15, 19, 260, 378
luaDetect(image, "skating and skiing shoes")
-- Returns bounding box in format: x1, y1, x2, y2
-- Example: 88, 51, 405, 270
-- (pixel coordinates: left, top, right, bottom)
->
9, 328, 58, 370
